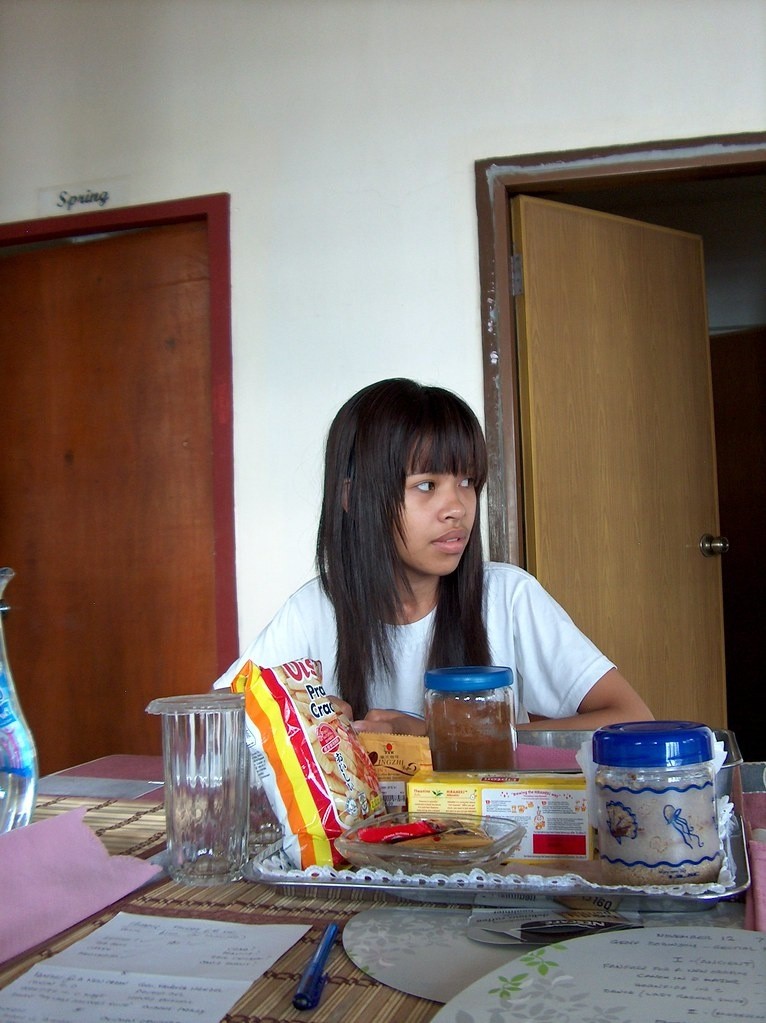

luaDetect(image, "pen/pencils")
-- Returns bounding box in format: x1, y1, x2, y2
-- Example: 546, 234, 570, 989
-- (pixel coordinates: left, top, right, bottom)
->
290, 921, 345, 1012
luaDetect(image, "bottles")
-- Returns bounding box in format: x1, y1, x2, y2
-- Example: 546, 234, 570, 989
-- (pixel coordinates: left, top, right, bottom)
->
424, 667, 520, 773
591, 721, 719, 887
0, 566, 40, 835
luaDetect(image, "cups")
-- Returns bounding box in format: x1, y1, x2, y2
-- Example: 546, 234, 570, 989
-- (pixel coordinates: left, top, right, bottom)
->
207, 687, 282, 855
145, 695, 251, 886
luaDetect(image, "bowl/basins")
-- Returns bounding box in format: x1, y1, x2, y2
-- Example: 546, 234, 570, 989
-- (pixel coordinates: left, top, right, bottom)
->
333, 811, 528, 875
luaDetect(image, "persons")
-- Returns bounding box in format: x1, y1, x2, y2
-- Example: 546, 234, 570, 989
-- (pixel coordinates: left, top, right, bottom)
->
209, 377, 656, 736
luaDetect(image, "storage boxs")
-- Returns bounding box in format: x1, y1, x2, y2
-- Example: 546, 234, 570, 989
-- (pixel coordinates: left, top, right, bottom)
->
405, 767, 595, 862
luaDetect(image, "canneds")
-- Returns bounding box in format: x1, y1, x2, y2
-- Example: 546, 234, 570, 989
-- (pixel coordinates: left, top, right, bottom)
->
588, 720, 720, 887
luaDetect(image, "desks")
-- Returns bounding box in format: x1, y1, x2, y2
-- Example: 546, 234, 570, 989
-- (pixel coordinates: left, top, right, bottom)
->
0, 751, 766, 1023
